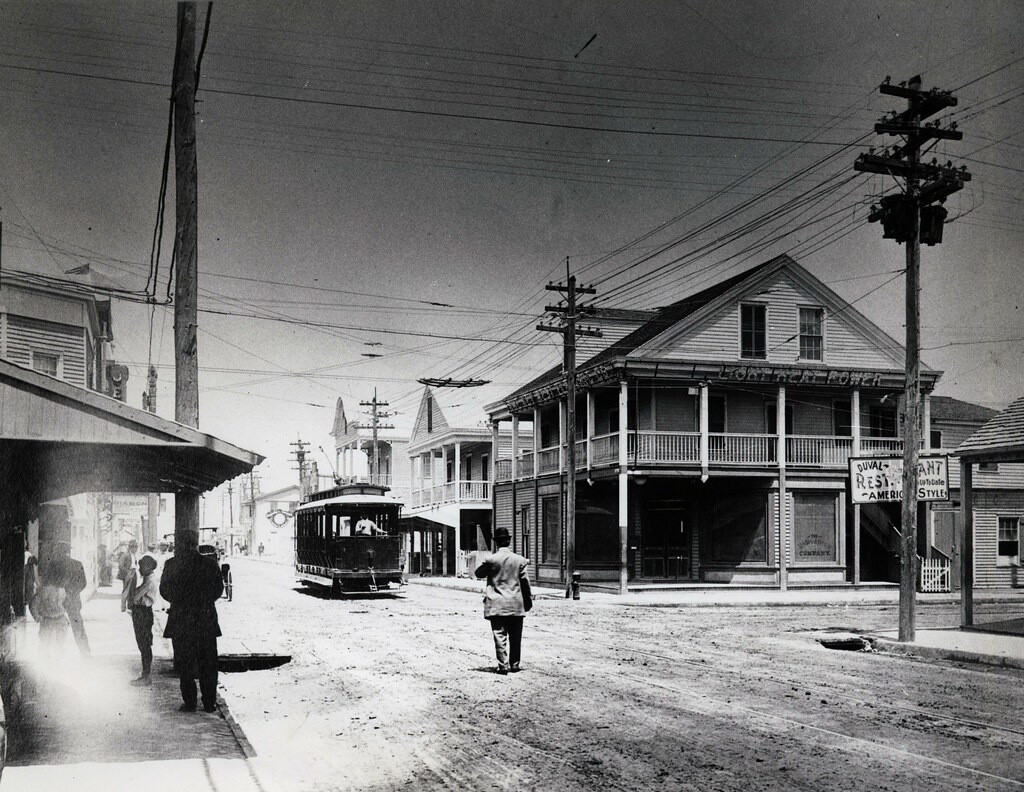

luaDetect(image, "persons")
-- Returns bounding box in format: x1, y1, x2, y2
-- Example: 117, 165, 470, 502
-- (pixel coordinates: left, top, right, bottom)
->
24, 539, 90, 657
99, 540, 175, 612
130, 556, 157, 687
475, 528, 532, 674
159, 530, 224, 712
355, 513, 388, 536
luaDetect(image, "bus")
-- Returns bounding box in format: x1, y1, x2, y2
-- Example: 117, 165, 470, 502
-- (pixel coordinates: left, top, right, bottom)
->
290, 482, 411, 596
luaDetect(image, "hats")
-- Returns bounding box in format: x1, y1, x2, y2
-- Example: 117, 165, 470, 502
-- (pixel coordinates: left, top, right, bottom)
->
493, 527, 512, 540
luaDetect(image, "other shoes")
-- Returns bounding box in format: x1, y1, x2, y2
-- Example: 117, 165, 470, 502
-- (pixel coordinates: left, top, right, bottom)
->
204, 704, 216, 713
511, 668, 519, 672
497, 667, 507, 675
131, 675, 151, 686
181, 704, 194, 713
